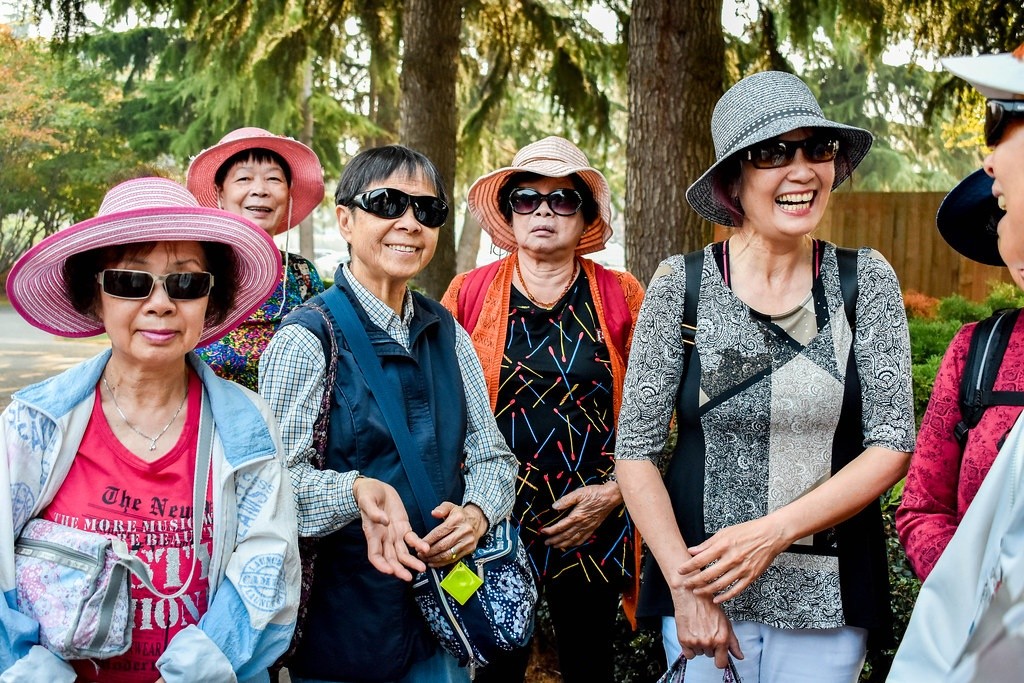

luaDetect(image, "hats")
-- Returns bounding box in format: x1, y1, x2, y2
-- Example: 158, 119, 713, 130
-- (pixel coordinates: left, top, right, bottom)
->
936, 168, 1008, 266
468, 136, 614, 257
685, 72, 874, 229
5, 178, 283, 349
941, 39, 1024, 97
187, 127, 324, 235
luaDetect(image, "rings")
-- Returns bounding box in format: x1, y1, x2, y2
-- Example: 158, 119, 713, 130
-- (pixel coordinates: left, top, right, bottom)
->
451, 548, 456, 559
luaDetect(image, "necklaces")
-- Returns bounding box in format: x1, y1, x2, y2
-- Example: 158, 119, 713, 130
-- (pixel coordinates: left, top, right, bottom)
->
515, 251, 578, 309
102, 370, 190, 451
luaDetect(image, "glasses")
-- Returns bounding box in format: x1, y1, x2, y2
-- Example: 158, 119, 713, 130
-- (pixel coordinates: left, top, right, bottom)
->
984, 96, 1024, 146
96, 269, 214, 299
733, 134, 839, 169
351, 188, 449, 229
508, 186, 589, 217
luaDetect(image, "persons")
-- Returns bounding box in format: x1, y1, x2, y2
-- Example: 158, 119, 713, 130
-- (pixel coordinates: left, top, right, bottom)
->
186, 126, 520, 683
613, 70, 915, 683
0, 176, 302, 683
438, 135, 679, 683
886, 39, 1024, 683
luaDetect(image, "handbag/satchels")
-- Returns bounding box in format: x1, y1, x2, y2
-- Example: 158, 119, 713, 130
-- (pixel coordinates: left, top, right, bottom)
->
13, 518, 133, 660
411, 515, 544, 667
